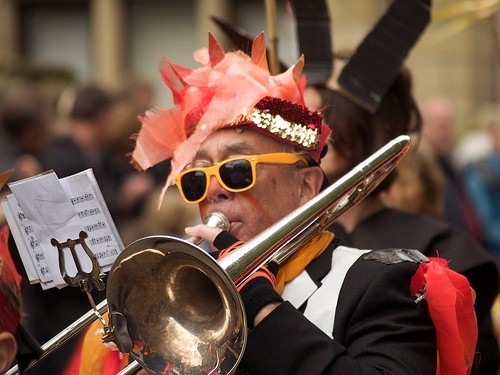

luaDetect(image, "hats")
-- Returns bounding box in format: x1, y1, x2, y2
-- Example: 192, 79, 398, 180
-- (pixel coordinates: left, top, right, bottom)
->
128, 31, 332, 212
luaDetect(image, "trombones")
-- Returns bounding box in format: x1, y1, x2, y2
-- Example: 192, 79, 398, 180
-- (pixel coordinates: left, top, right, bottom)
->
3, 135, 413, 375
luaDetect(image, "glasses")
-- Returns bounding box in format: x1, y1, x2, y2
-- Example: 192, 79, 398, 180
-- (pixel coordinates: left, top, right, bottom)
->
173, 152, 309, 204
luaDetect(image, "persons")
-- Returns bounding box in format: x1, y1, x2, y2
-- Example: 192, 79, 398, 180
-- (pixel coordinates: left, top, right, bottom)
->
170, 83, 477, 375
0, 65, 213, 375
286, 49, 500, 375
